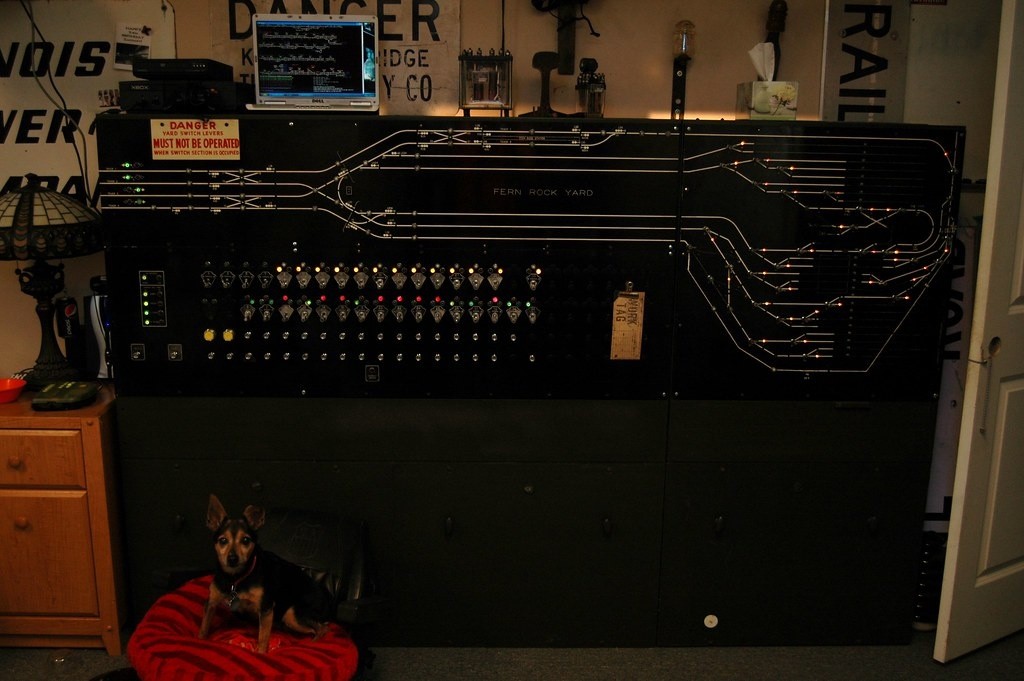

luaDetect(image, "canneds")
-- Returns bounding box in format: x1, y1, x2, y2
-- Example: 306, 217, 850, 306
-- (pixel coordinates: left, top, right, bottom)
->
55, 296, 81, 338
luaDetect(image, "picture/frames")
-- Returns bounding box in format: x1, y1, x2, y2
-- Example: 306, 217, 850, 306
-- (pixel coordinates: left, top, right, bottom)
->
458, 60, 512, 110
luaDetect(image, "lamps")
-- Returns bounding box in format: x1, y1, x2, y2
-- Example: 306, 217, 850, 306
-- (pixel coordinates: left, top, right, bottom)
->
0, 173, 104, 393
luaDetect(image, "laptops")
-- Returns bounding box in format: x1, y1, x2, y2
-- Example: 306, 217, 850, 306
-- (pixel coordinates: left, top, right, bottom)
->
246, 14, 379, 112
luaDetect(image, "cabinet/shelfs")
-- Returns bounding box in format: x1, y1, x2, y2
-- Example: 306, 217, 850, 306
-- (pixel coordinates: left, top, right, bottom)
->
114, 398, 670, 649
654, 401, 937, 649
0, 387, 124, 657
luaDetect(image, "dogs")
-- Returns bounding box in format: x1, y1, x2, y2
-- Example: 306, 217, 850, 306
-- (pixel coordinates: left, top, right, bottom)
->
198, 492, 333, 654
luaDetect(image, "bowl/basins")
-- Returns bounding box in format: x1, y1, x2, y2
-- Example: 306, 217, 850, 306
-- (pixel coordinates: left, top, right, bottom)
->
0, 378, 27, 403
